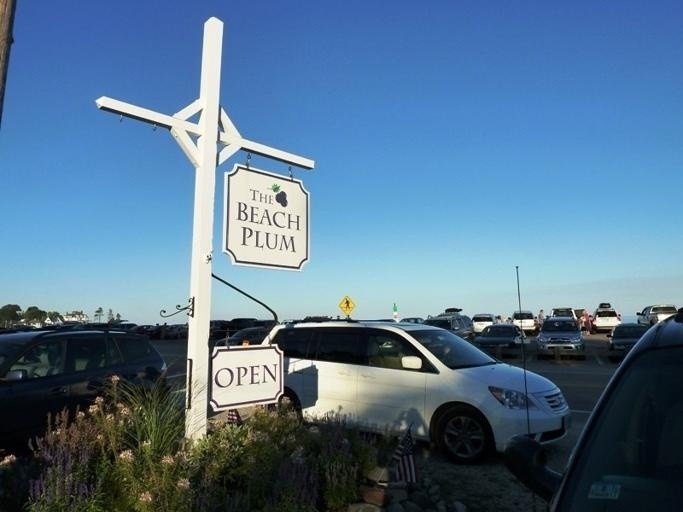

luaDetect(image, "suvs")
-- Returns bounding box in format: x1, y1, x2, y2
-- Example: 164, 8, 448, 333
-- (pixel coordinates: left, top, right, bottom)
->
422, 308, 477, 345
534, 317, 587, 361
588, 303, 623, 332
261, 318, 575, 464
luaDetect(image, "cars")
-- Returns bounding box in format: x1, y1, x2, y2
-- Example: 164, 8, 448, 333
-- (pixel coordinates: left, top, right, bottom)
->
605, 322, 649, 363
398, 317, 425, 323
210, 314, 278, 348
550, 306, 591, 329
507, 311, 539, 335
549, 308, 683, 511
0, 321, 187, 463
473, 324, 530, 358
471, 313, 499, 332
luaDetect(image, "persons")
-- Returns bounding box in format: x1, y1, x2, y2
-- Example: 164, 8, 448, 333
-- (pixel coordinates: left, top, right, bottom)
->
533, 323, 541, 338
533, 316, 538, 326
537, 309, 546, 327
577, 309, 595, 336
493, 314, 513, 325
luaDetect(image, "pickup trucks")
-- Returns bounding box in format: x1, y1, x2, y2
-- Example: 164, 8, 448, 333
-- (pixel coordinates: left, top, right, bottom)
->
636, 305, 679, 327
